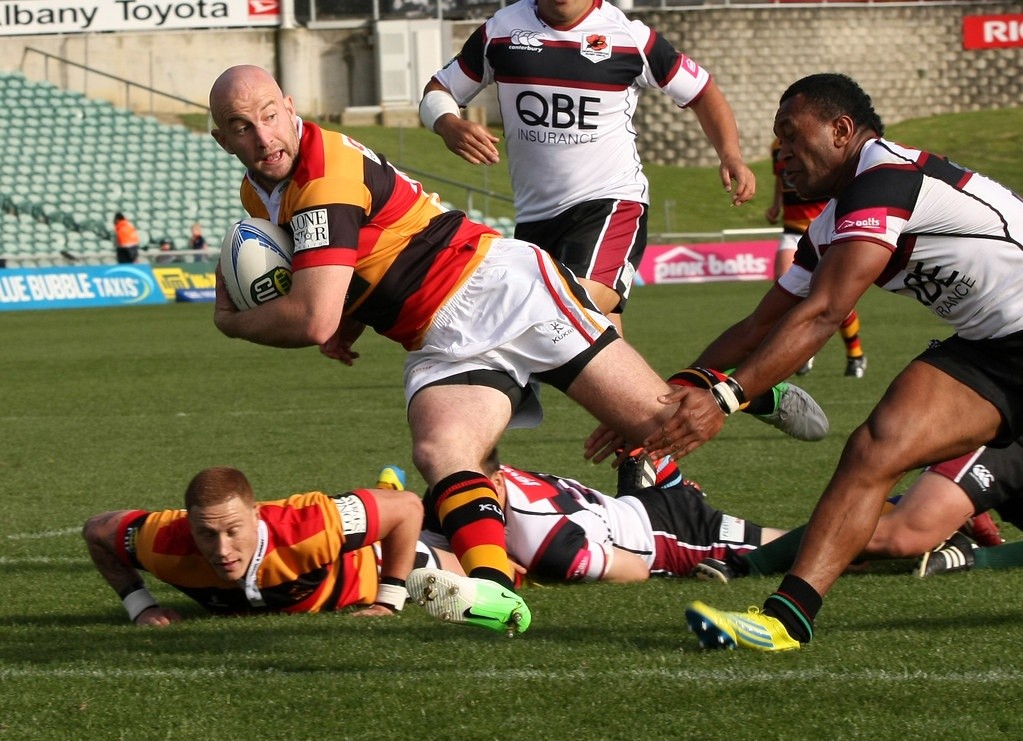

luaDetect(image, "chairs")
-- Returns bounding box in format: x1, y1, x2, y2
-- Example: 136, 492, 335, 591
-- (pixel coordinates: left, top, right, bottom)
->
0, 71, 515, 269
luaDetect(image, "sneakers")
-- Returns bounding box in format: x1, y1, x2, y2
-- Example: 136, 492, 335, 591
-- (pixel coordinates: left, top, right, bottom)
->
722, 368, 829, 441
614, 453, 657, 499
957, 510, 1004, 549
405, 568, 531, 639
379, 465, 406, 491
845, 355, 867, 377
794, 355, 814, 376
915, 531, 977, 580
685, 600, 800, 655
696, 558, 732, 587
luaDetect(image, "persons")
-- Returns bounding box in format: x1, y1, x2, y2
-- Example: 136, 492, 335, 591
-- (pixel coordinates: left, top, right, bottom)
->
582, 73, 1022, 649
81, 465, 467, 628
479, 445, 803, 584
115, 213, 207, 264
418, 1, 756, 493
212, 64, 829, 634
695, 436, 1022, 577
767, 123, 867, 378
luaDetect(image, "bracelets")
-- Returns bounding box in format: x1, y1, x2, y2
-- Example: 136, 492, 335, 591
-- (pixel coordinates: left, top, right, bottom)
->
123, 588, 159, 622
373, 576, 407, 613
710, 376, 748, 416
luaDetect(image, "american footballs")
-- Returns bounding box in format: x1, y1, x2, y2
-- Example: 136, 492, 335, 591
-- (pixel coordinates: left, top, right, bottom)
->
218, 216, 300, 314
